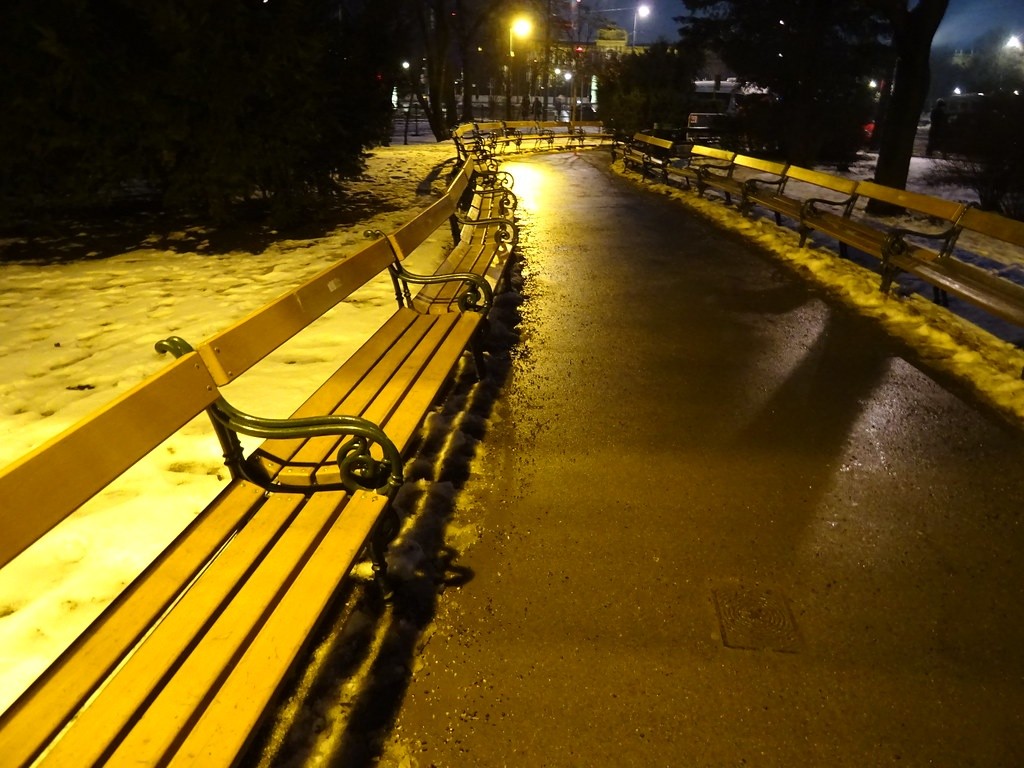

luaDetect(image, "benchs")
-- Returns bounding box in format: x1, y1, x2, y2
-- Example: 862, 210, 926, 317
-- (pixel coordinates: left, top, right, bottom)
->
0, 113, 1023, 768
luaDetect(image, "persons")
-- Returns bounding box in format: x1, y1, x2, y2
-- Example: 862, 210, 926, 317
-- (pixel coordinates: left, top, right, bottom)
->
532, 97, 542, 121
521, 95, 530, 121
925, 99, 950, 159
554, 98, 562, 121
